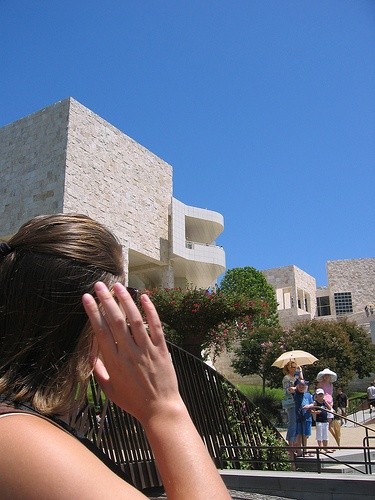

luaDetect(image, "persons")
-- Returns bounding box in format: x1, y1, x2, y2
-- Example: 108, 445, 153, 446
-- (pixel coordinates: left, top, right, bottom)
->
367, 382, 375, 414
282, 361, 349, 457
1, 213, 233, 500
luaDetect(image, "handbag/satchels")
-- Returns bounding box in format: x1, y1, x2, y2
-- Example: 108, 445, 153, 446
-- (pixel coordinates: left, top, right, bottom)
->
282, 393, 295, 409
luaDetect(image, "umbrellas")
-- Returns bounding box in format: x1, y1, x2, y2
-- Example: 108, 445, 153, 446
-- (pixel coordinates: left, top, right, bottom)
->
271, 349, 318, 369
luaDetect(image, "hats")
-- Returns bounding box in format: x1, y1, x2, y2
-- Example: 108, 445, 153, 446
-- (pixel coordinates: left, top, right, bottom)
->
316, 389, 324, 395
317, 369, 337, 382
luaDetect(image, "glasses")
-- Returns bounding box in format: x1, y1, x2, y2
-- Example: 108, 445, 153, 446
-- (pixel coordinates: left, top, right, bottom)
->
126, 287, 138, 305
